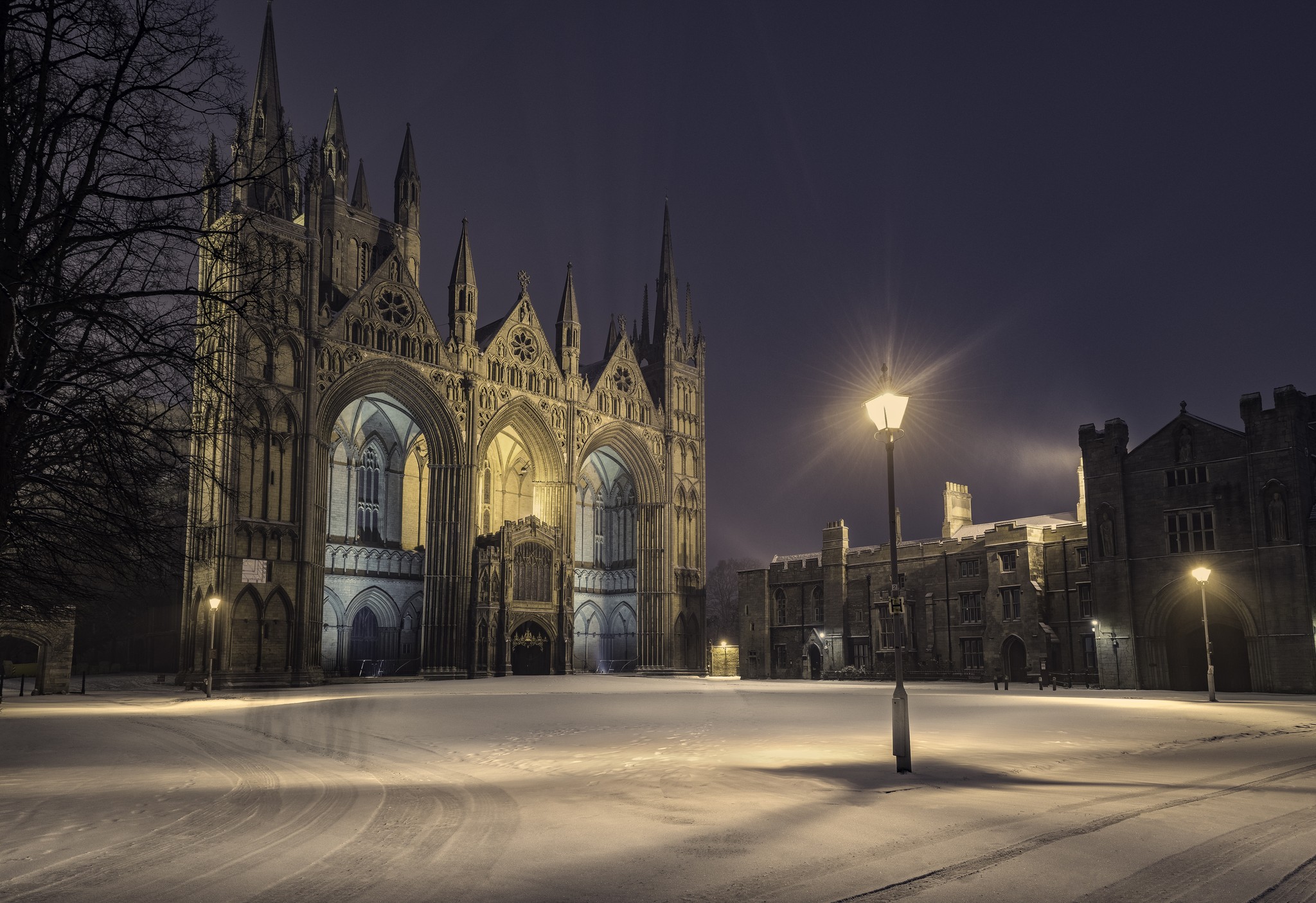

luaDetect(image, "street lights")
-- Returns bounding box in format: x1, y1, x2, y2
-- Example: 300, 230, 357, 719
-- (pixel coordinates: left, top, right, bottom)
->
859, 364, 912, 774
1192, 558, 1215, 702
207, 589, 222, 697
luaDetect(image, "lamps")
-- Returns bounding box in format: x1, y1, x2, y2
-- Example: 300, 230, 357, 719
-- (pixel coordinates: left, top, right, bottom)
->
824, 646, 828, 655
721, 642, 727, 652
1092, 628, 1095, 632
1092, 621, 1097, 624
